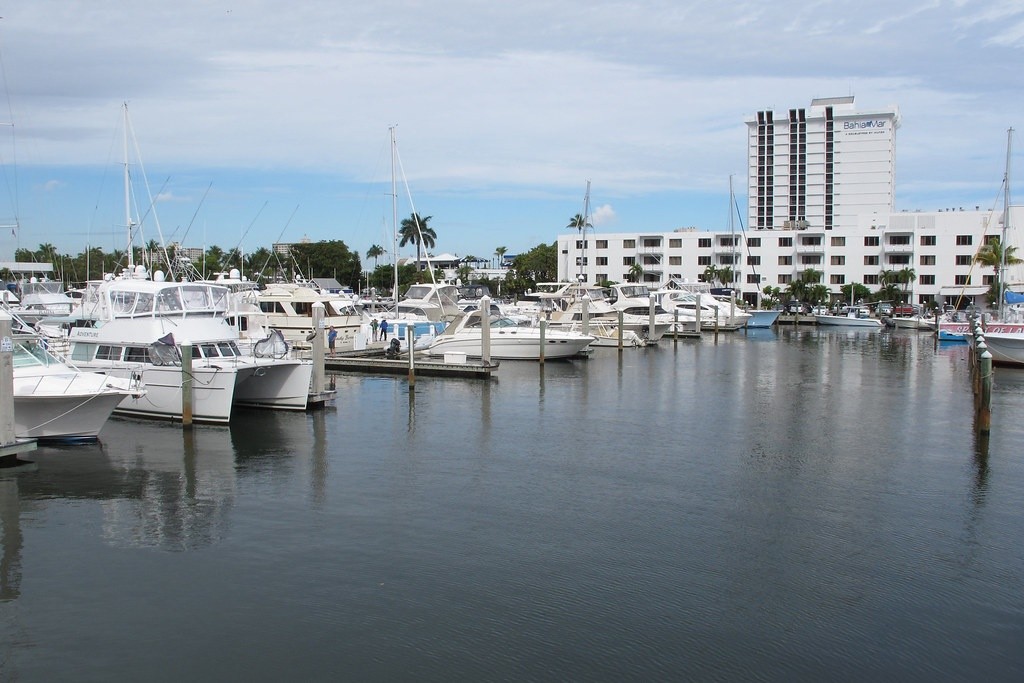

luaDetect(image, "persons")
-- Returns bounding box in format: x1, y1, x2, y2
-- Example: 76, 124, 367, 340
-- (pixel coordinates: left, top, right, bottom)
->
380, 320, 388, 340
370, 319, 379, 341
327, 326, 338, 357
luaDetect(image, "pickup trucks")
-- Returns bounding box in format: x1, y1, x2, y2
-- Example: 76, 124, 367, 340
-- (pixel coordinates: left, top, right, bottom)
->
789, 304, 807, 316
833, 303, 849, 317
896, 304, 914, 317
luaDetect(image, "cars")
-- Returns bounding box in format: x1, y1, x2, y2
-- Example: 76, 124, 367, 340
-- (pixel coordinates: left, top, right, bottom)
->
773, 305, 785, 315
964, 306, 981, 318
856, 306, 870, 317
874, 303, 894, 318
812, 306, 829, 316
941, 305, 958, 322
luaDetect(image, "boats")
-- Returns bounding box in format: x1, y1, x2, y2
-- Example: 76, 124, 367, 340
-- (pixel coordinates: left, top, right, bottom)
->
814, 315, 885, 327
963, 332, 1024, 363
893, 318, 931, 328
0, 100, 785, 457
